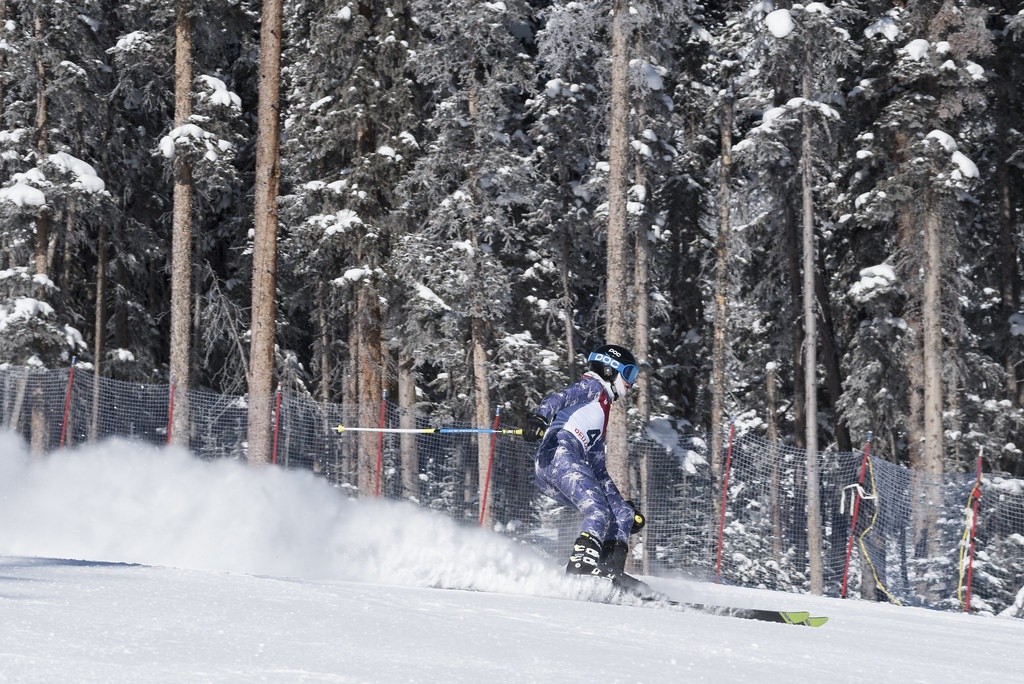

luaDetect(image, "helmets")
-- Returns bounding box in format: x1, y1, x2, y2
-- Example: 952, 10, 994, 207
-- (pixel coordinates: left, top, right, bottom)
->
588, 345, 638, 381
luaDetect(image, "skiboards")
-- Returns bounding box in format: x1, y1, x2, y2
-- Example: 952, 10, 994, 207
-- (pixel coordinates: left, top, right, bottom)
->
427, 575, 831, 630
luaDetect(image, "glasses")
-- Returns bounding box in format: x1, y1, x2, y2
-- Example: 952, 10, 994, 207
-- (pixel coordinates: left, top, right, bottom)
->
620, 364, 639, 386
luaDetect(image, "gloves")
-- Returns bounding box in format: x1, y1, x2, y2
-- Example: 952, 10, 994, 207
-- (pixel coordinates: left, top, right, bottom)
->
626, 499, 645, 534
521, 413, 548, 443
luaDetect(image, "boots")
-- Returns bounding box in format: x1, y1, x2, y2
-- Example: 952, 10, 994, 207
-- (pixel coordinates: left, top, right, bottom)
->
566, 531, 612, 583
598, 539, 649, 599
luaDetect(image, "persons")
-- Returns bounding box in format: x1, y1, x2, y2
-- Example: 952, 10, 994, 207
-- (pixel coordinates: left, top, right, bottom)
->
520, 345, 651, 599
